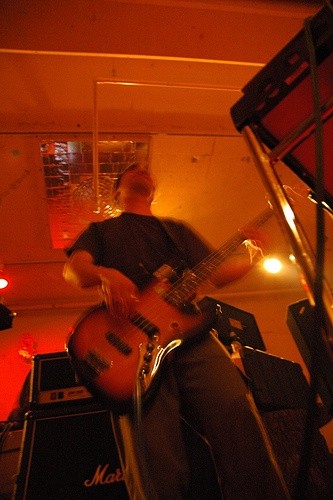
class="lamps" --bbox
[40,134,154,253]
[0,277,9,291]
[262,258,282,275]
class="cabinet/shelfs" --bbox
[196,296,268,350]
[187,344,332,500]
[10,351,133,500]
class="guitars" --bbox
[66,208,275,411]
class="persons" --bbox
[59,163,291,500]
